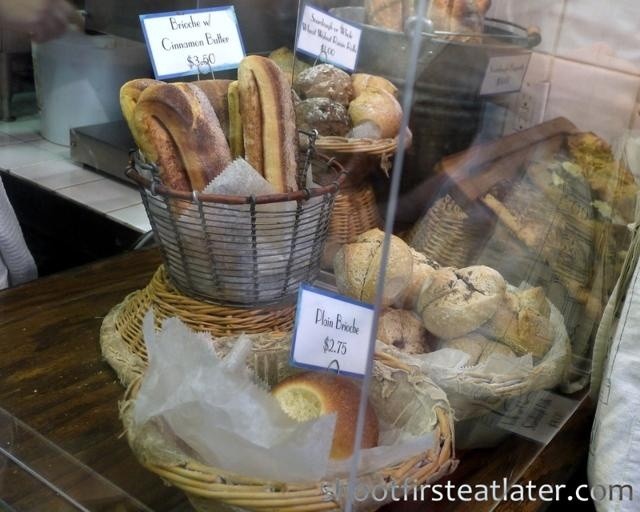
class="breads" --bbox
[119,46,640,367]
[267,372,379,459]
[364,0,492,42]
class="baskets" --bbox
[101,131,630,512]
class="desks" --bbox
[1,117,608,511]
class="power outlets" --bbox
[502,78,551,138]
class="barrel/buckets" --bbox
[328,6,543,201]
[30,32,157,147]
